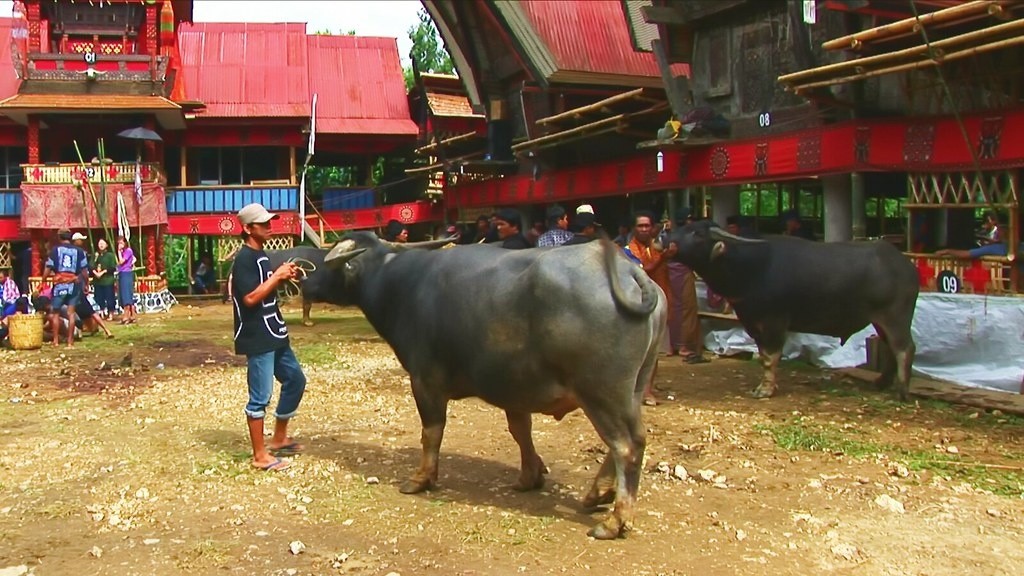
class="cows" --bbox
[301,229,671,543]
[660,217,922,406]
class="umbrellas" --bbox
[116,126,163,159]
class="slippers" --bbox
[682,353,711,364]
[273,438,304,451]
[252,458,291,471]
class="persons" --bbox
[231,202,307,471]
[936,210,1024,266]
[384,204,814,407]
[0,229,218,346]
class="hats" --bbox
[575,212,602,226]
[577,205,594,215]
[237,203,280,224]
[71,232,88,240]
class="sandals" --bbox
[116,319,137,325]
